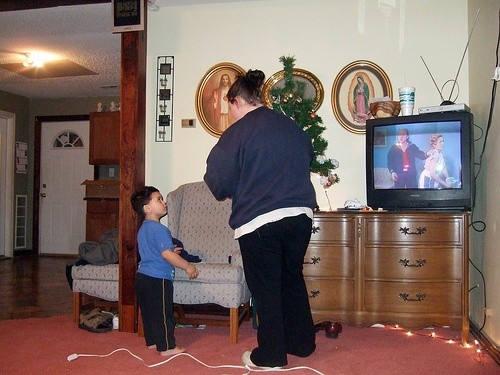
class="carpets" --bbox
[0,313,500,375]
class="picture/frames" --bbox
[331,60,393,134]
[195,62,247,138]
[262,69,324,113]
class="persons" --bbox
[131,186,199,356]
[387,128,452,189]
[203,69,320,369]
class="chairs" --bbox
[71,264,119,323]
[138,181,252,345]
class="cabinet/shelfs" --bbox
[86,201,120,244]
[16,196,27,250]
[254,211,471,346]
[88,112,120,164]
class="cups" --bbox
[398,86,416,116]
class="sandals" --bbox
[79,308,113,333]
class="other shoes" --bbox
[242,350,277,367]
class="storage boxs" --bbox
[80,180,120,198]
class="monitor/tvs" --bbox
[366,111,476,213]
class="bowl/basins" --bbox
[370,101,401,119]
